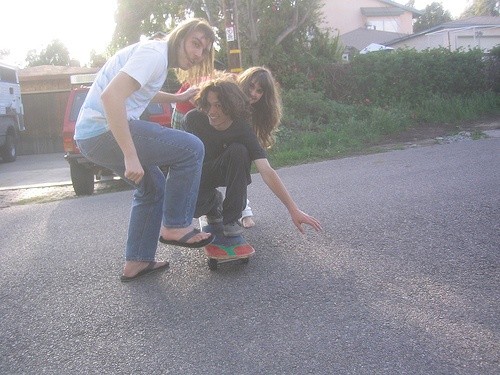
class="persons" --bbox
[73,19,217,282]
[169,67,322,237]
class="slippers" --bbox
[159,227,215,248]
[121,260,169,282]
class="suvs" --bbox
[0,64,28,162]
[60,72,175,195]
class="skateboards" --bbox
[199,211,256,271]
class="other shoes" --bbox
[237,199,255,228]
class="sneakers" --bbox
[207,192,224,224]
[223,222,244,237]
